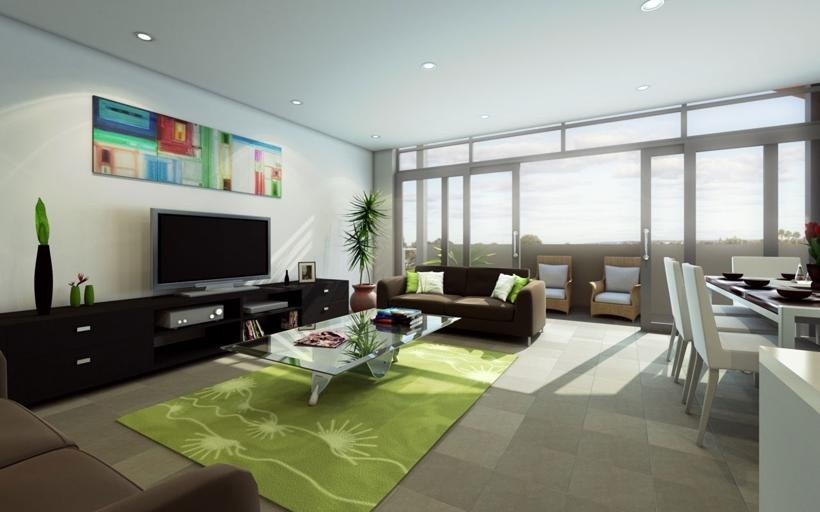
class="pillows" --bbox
[405,271,418,293]
[416,271,444,295]
[508,274,530,303]
[491,273,518,303]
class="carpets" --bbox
[115,340,518,512]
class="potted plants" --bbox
[337,188,392,312]
[68,273,88,305]
[33,199,52,311]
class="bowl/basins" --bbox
[722,273,812,299]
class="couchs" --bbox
[0,352,261,512]
[377,266,546,346]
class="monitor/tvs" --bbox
[149,206,273,299]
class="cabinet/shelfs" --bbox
[0,279,350,408]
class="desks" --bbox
[219,307,462,405]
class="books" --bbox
[243,319,265,340]
[376,307,423,328]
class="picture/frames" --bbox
[298,261,317,283]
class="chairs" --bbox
[586,256,642,322]
[663,256,819,450]
[534,255,573,316]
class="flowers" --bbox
[805,221,820,263]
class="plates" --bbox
[717,278,820,303]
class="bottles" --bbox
[284,270,290,285]
